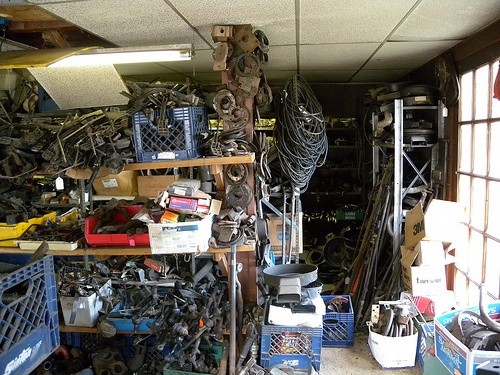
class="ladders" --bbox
[352,172,382,262]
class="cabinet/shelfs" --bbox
[0,152,255,335]
[208,123,366,248]
[371,97,446,299]
[186,167,300,274]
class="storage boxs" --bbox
[133,175,222,255]
[133,105,206,162]
[367,196,500,375]
[264,213,305,255]
[333,209,364,222]
[84,201,149,248]
[320,295,355,349]
[261,299,322,375]
[0,253,60,375]
[92,171,139,196]
[1,211,57,247]
[61,278,223,375]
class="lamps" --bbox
[48,43,197,64]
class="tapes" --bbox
[257,219,267,240]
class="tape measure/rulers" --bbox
[261,193,296,226]
